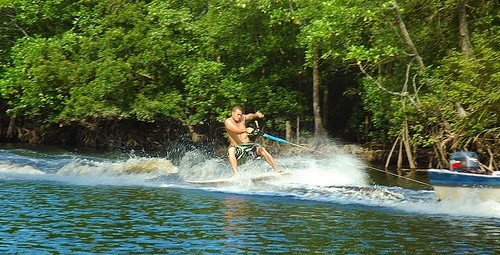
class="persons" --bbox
[224,106,283,178]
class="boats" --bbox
[426,151,500,212]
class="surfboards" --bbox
[184,171,291,183]
